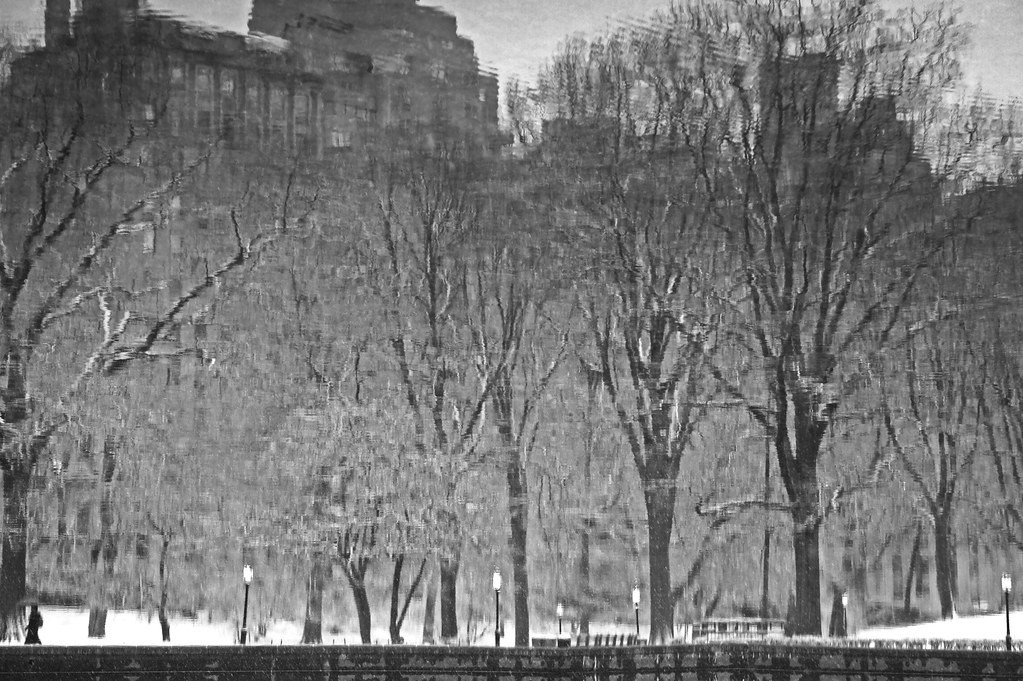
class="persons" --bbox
[24,606,43,644]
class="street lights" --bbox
[557,603,563,634]
[493,571,502,647]
[1001,571,1012,651]
[240,564,253,643]
[632,588,641,635]
[841,592,848,636]
